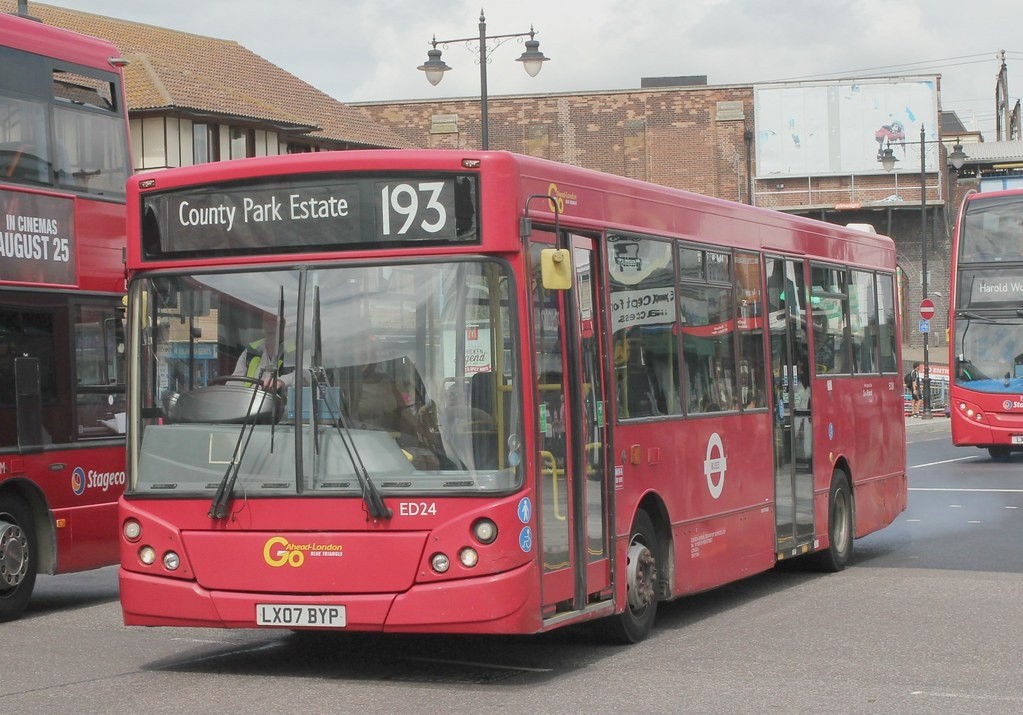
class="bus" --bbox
[1,17,160,625]
[946,186,1023,461]
[114,142,912,649]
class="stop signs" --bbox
[919,298,936,320]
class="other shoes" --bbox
[911,413,920,418]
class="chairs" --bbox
[469,371,509,444]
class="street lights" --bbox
[416,9,550,150]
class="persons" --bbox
[795,306,844,374]
[545,394,567,458]
[225,291,315,393]
[911,361,923,419]
[675,383,786,468]
[439,383,470,425]
[983,343,1010,367]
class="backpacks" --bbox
[904,370,916,389]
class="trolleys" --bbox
[927,376,949,415]
